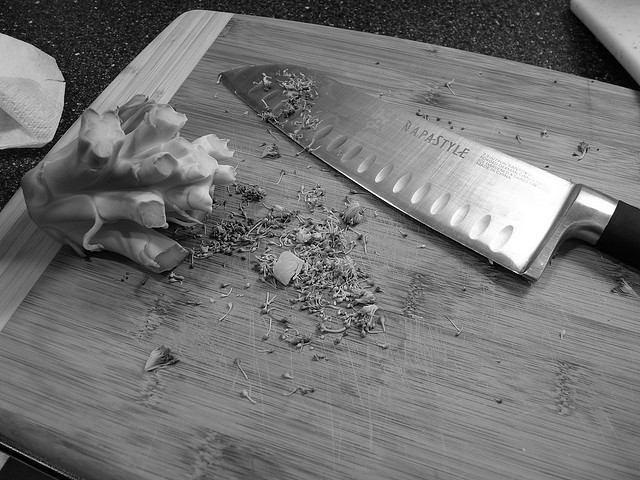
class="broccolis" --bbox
[20,94,238,274]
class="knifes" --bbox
[218,64,640,283]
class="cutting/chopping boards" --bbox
[0,7,639,476]
